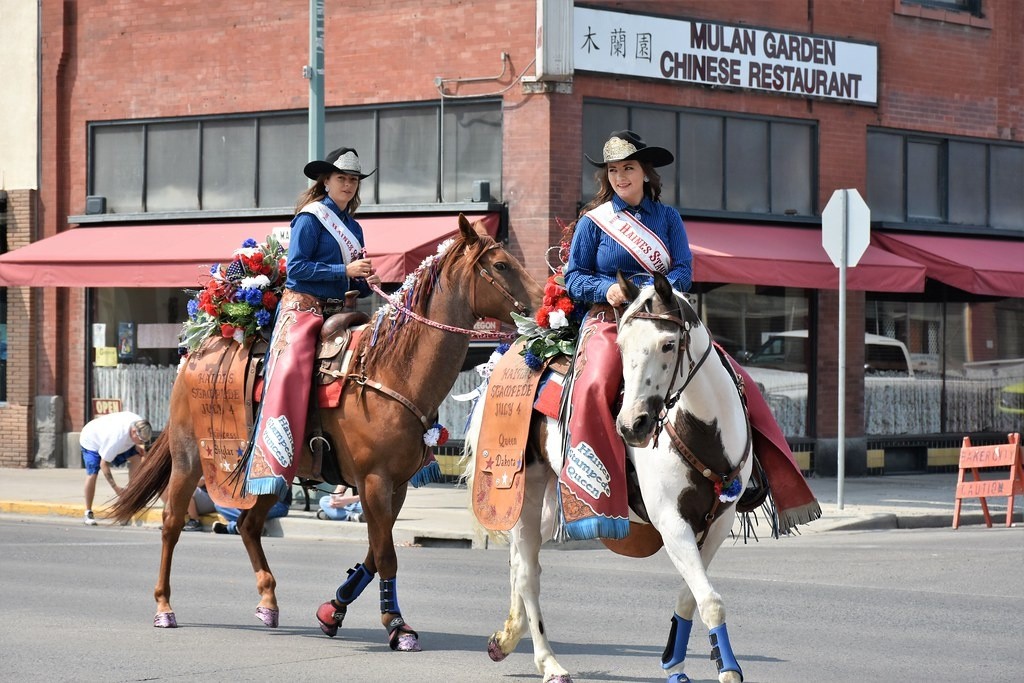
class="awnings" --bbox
[682,218,927,291]
[0,213,499,287]
[869,230,1024,298]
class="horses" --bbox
[458,270,753,683]
[94,213,545,652]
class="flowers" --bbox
[493,214,580,369]
[180,235,289,355]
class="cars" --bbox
[992,378,1024,428]
[733,328,917,411]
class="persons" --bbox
[247,146,382,503]
[80,411,152,524]
[557,130,822,541]
[182,475,216,531]
[316,485,367,522]
[212,486,293,534]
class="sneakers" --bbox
[235,525,241,535]
[158,525,164,530]
[181,519,202,531]
[211,521,228,534]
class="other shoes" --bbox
[84,510,97,525]
[316,509,330,521]
[352,513,367,523]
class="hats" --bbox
[587,129,674,168]
[302,147,378,181]
